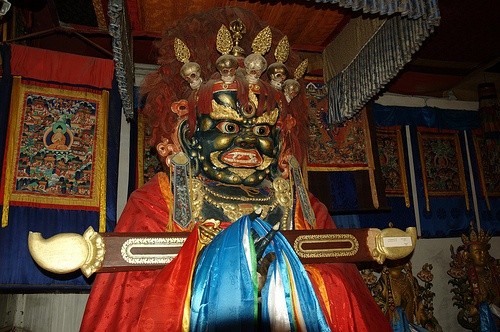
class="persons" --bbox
[361,227,500,332]
[79,7,391,332]
